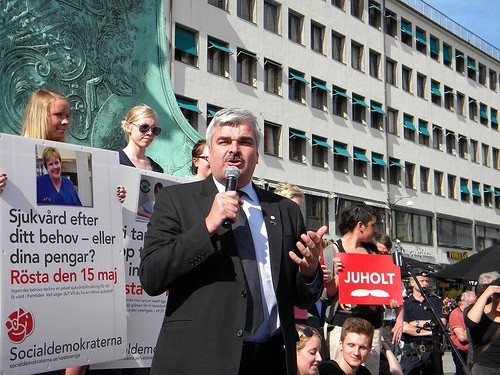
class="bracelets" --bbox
[416,320,419,327]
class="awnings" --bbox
[175,24,430,137]
[290,131,404,169]
[369,1,477,71]
[460,179,500,197]
[431,81,498,125]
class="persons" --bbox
[295,323,322,375]
[307,205,407,375]
[190,139,212,181]
[400,268,447,375]
[139,108,328,375]
[119,104,164,174]
[275,182,304,209]
[37,147,82,207]
[318,317,374,375]
[137,182,163,219]
[429,271,500,375]
[0,89,126,375]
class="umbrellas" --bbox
[431,238,500,286]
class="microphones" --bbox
[222,166,241,229]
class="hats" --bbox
[411,268,428,277]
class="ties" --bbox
[231,191,264,335]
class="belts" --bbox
[243,336,284,354]
[407,344,434,356]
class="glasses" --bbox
[131,122,162,136]
[378,250,391,255]
[299,326,318,338]
